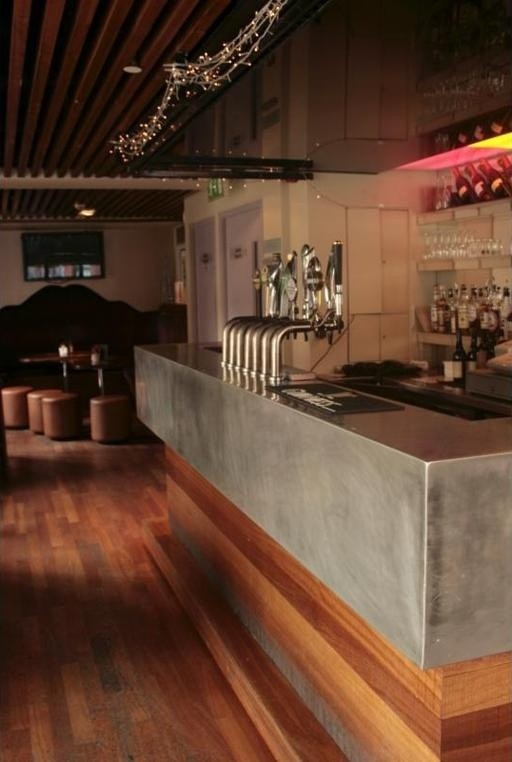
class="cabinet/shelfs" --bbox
[416,159,512,402]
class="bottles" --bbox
[455,104,512,148]
[431,280,510,332]
[451,331,480,384]
[452,155,511,203]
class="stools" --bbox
[41,394,81,440]
[90,395,130,443]
[1,386,33,429]
[26,389,61,433]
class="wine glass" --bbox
[433,175,452,211]
[422,229,477,258]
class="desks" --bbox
[60,356,123,395]
[19,351,90,393]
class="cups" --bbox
[441,360,455,383]
[433,132,453,155]
[468,236,503,256]
